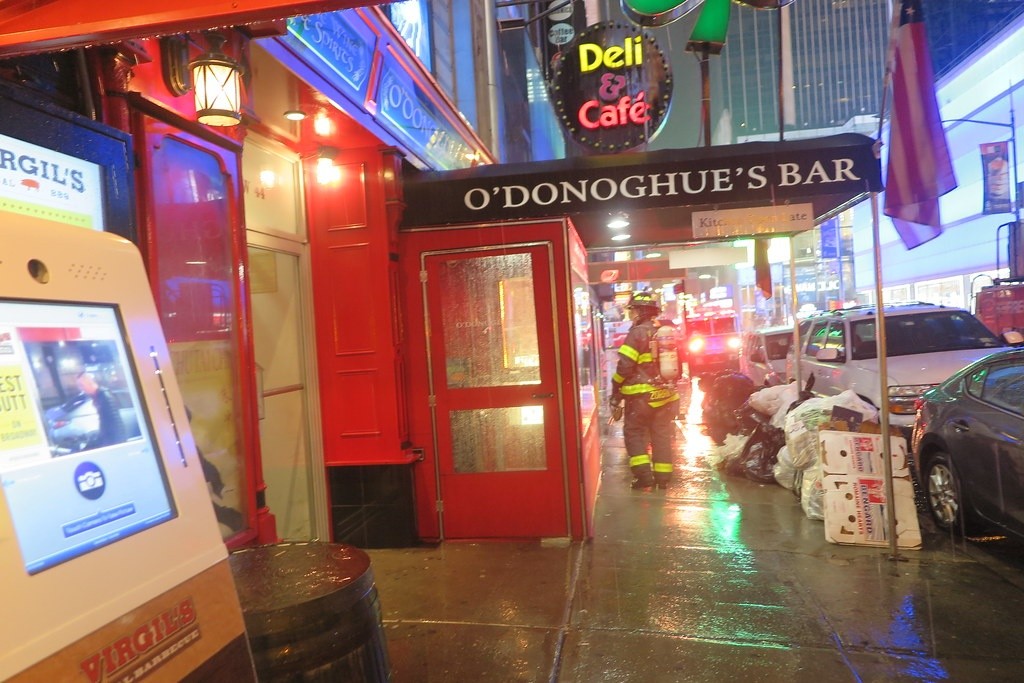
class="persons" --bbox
[609,293,680,489]
[74,371,127,451]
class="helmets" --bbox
[624,292,659,308]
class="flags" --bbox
[753,236,773,300]
[884,0,957,251]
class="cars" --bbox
[909,345,1024,538]
[739,326,795,387]
[786,301,1016,449]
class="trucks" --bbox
[684,312,742,375]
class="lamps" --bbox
[160,30,242,127]
[316,146,339,186]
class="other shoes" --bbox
[632,478,654,487]
[655,480,670,489]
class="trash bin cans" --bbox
[229,540,391,683]
